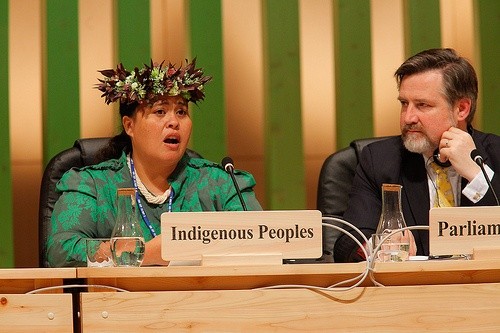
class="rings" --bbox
[446,140,448,147]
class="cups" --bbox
[86,238,111,267]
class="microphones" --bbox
[470,149,500,206]
[221,156,247,211]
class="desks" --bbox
[0,258,500,333]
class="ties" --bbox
[430,161,456,208]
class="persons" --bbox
[45,59,263,267]
[333,48,500,263]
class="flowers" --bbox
[95,57,214,106]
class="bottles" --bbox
[375,183,411,263]
[109,187,145,268]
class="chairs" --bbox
[317,136,394,261]
[38,137,204,268]
